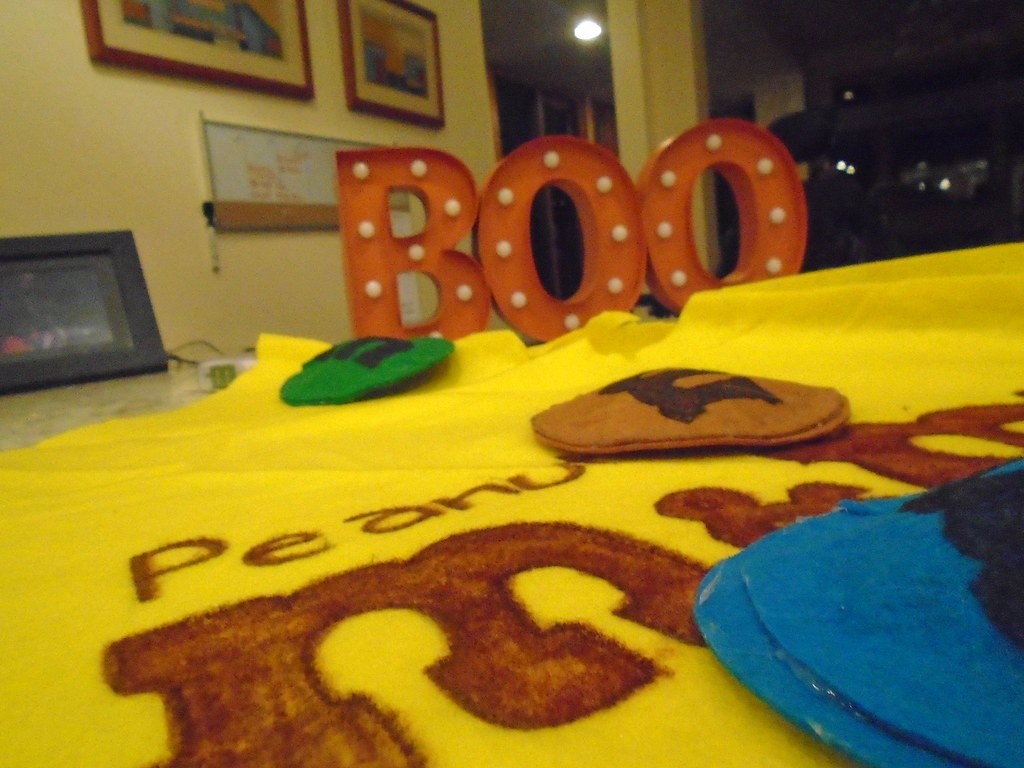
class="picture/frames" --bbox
[79,0,318,104]
[0,229,171,394]
[336,0,446,129]
